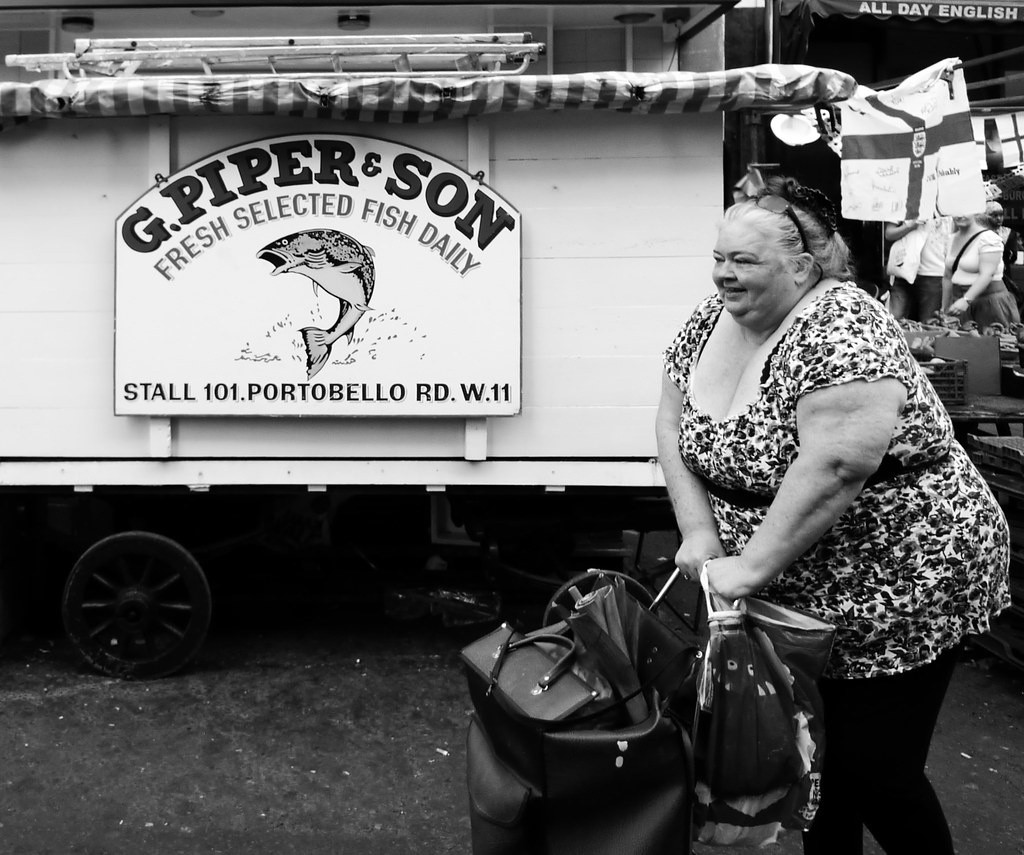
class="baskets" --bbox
[911,356,967,405]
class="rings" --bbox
[683,573,692,580]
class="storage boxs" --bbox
[911,352,969,403]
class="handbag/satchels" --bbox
[460,558,836,855]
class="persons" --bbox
[885,201,1024,330]
[654,174,1011,855]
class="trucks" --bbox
[0,33,856,679]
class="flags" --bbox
[838,57,987,220]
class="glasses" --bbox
[745,192,809,253]
